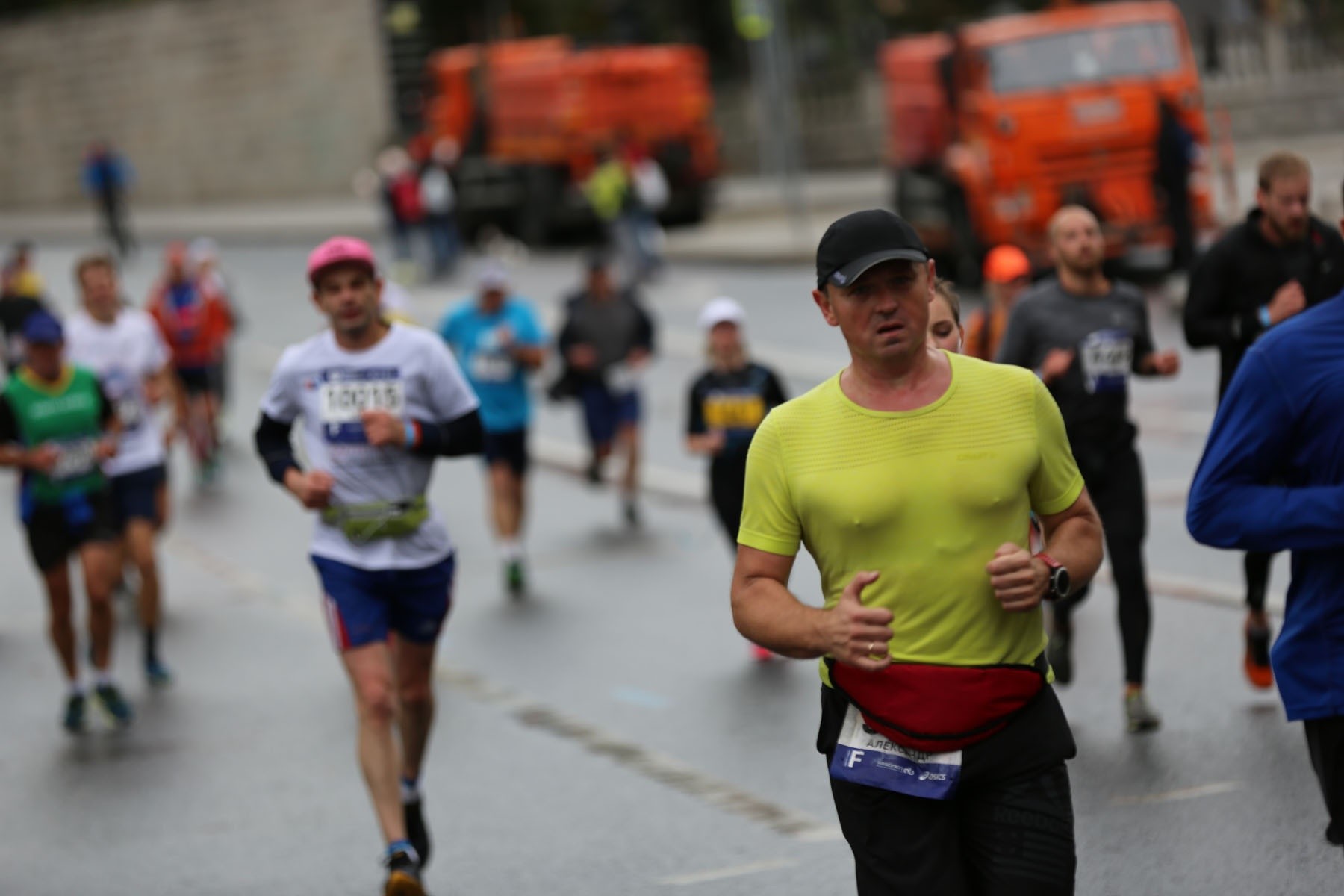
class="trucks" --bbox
[873,1,1222,292]
[411,33,720,251]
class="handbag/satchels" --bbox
[324,496,433,543]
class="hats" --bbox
[309,236,373,286]
[814,207,930,294]
[984,246,1030,285]
[701,298,744,333]
[24,314,62,345]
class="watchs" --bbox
[1036,551,1071,602]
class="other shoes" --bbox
[382,853,430,896]
[1244,619,1273,685]
[97,685,126,722]
[144,657,164,679]
[63,692,84,730]
[507,562,524,593]
[1047,628,1075,684]
[585,461,602,482]
[1123,690,1157,732]
[623,501,639,521]
[403,796,428,865]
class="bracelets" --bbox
[411,421,422,449]
[405,419,414,446]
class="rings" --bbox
[869,641,875,653]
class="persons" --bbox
[0,95,1343,896]
